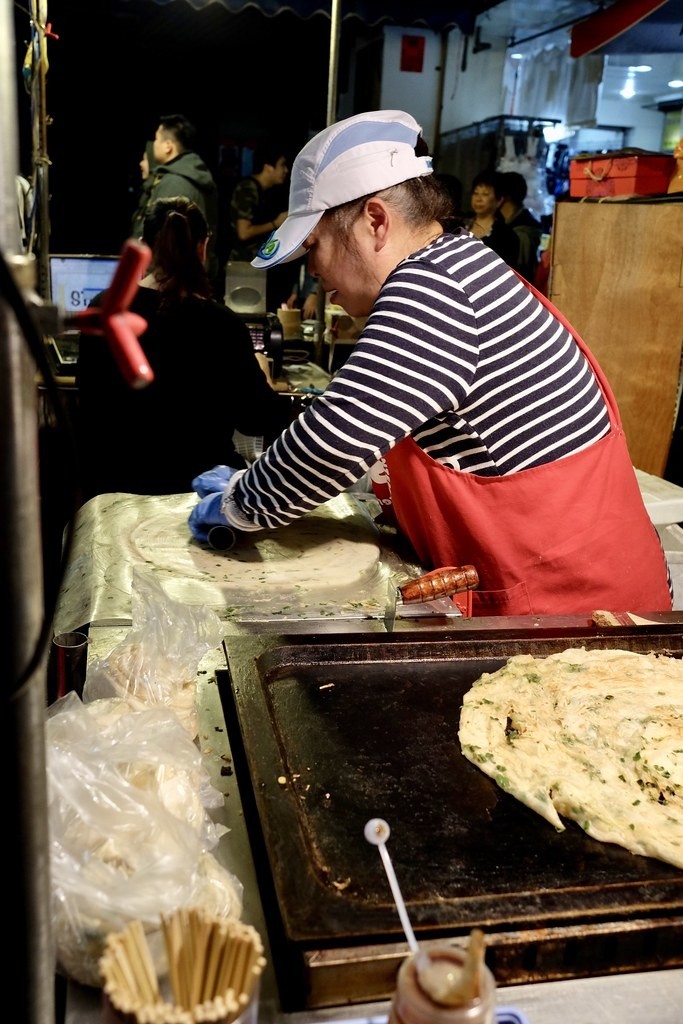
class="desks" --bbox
[33,338,333,452]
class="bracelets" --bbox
[271,221,277,229]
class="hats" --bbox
[250,109,433,271]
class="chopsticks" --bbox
[98,906,267,1024]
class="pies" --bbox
[458,648,683,870]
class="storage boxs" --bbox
[569,156,677,198]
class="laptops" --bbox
[46,254,124,367]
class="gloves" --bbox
[193,464,238,497]
[189,492,233,542]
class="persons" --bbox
[502,172,540,284]
[459,171,519,270]
[131,113,217,291]
[286,254,320,319]
[190,111,675,619]
[78,196,313,494]
[223,149,289,261]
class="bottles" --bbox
[388,945,496,1024]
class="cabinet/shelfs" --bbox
[546,194,683,478]
